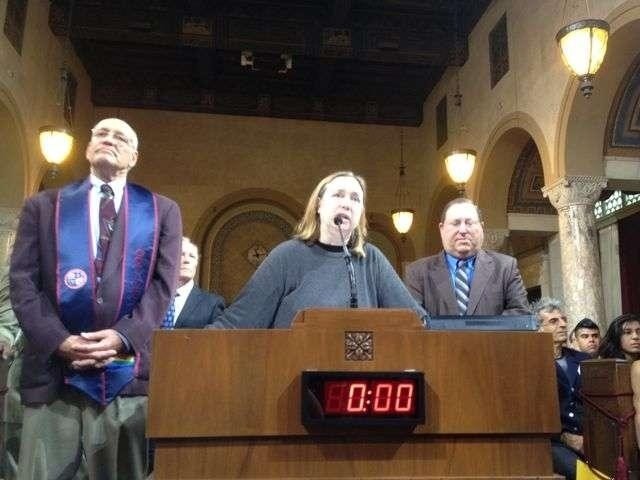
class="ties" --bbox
[93,185,117,290]
[454,258,470,318]
[162,292,178,329]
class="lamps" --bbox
[389,125,416,244]
[36,1,75,178]
[556,1,609,99]
[444,63,477,199]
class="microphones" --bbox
[334,215,358,308]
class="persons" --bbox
[7,116,184,479]
[203,171,428,330]
[403,197,533,316]
[158,236,225,329]
[574,317,601,357]
[529,295,591,480]
[568,330,581,352]
[598,312,640,361]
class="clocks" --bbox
[243,242,271,268]
